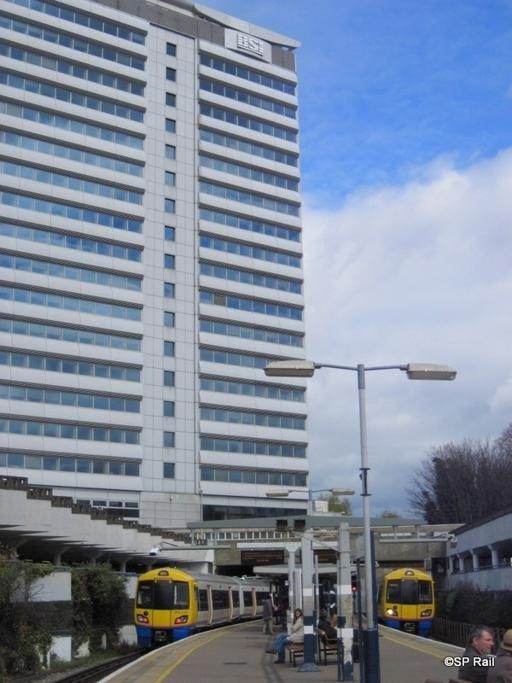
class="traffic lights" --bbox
[351,573,360,593]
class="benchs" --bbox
[449,678,472,683]
[316,628,337,665]
[287,645,304,666]
[425,679,445,683]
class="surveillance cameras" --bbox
[150,548,160,556]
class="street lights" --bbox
[261,356,460,682]
[264,484,356,672]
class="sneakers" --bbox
[265,649,276,654]
[274,659,285,664]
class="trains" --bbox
[132,565,280,653]
[377,565,439,640]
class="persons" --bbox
[318,611,338,646]
[263,594,278,635]
[458,625,494,683]
[486,629,512,683]
[265,608,304,663]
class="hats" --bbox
[499,628,512,651]
